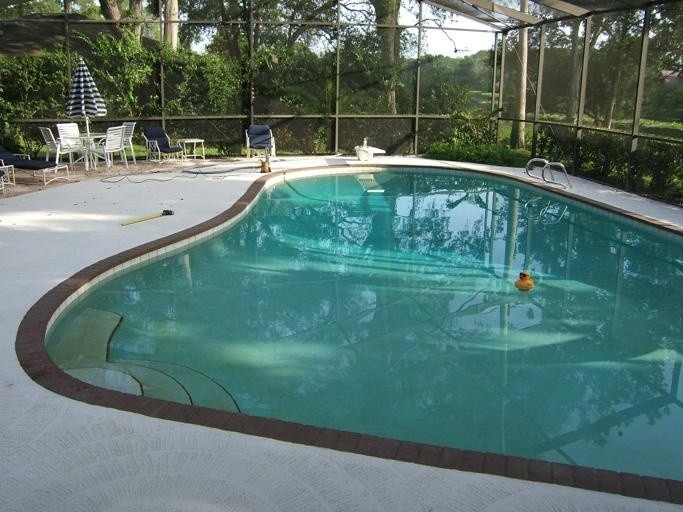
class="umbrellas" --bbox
[65,57,107,137]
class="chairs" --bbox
[245,124,276,160]
[37,118,183,168]
[1,146,69,195]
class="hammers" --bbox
[120,210,174,226]
